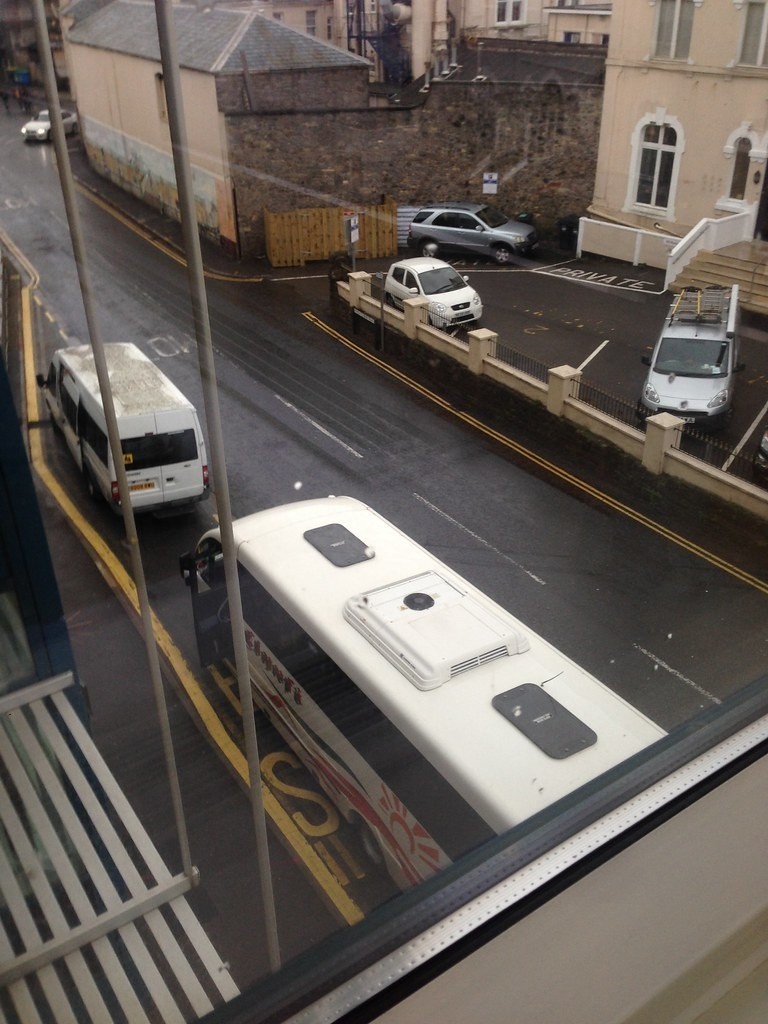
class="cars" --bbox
[383,257,483,328]
[21,109,79,143]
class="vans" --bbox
[36,340,211,516]
[637,284,745,433]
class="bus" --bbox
[178,495,668,895]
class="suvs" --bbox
[408,201,540,265]
